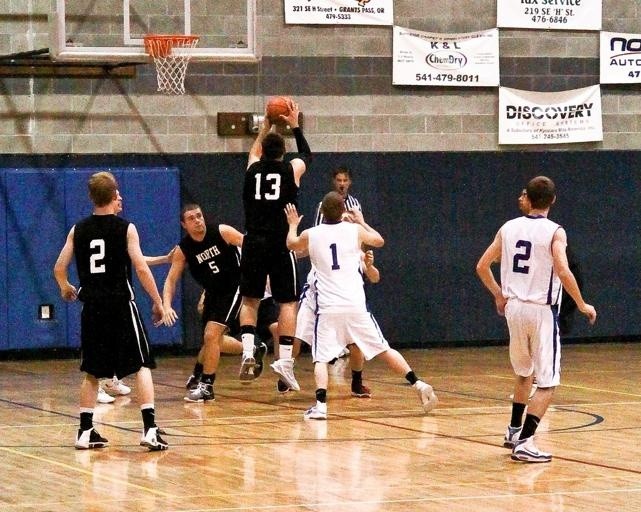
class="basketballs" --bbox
[266,97,292,125]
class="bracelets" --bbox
[492,288,503,297]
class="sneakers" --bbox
[504,424,552,463]
[411,380,438,412]
[351,386,372,398]
[140,424,169,450]
[239,342,301,392]
[96,376,131,403]
[304,407,327,420]
[184,375,215,403]
[75,426,110,449]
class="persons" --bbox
[284,191,440,420]
[93,190,177,402]
[237,100,313,390]
[509,187,586,400]
[476,175,598,463]
[54,172,168,452]
[315,168,363,365]
[163,203,379,402]
